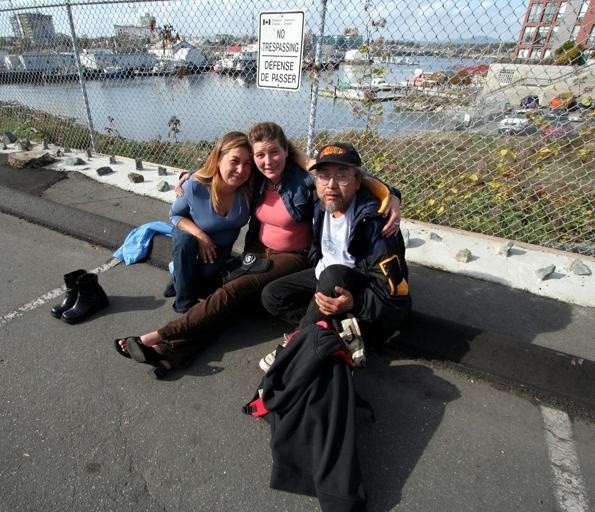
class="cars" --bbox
[495,91,594,136]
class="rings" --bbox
[394,222,400,229]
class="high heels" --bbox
[114,336,175,380]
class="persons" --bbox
[260,141,408,377]
[113,122,402,380]
[164,131,254,313]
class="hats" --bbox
[309,143,360,171]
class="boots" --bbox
[50,269,86,316]
[61,273,109,324]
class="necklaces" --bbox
[267,179,282,192]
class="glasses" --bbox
[315,173,353,186]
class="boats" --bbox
[1,35,486,98]
[316,47,409,103]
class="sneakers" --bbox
[259,343,286,373]
